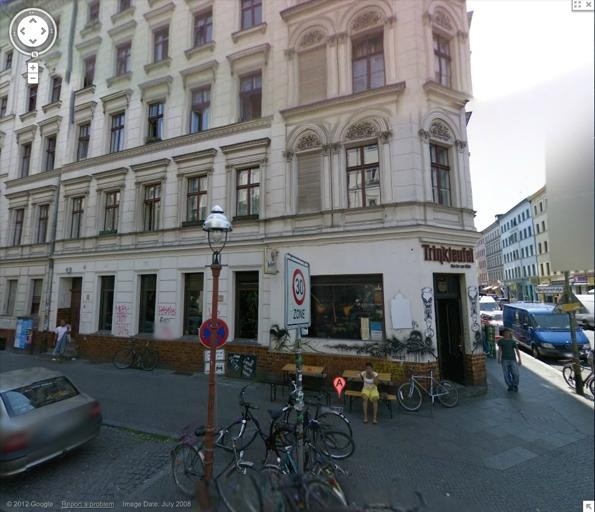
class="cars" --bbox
[0,366,103,479]
[571,308,595,329]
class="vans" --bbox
[478,295,505,333]
[503,301,591,362]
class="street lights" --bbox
[201,203,233,512]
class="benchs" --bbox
[343,389,397,410]
[262,379,317,402]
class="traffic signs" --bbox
[283,252,312,329]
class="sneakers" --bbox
[514,385,518,392]
[508,386,513,391]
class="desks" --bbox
[341,369,393,410]
[280,363,325,403]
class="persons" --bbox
[346,361,383,425]
[496,329,522,393]
[49,318,77,363]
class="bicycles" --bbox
[170,381,356,512]
[396,367,458,412]
[111,340,159,371]
[563,355,595,396]
